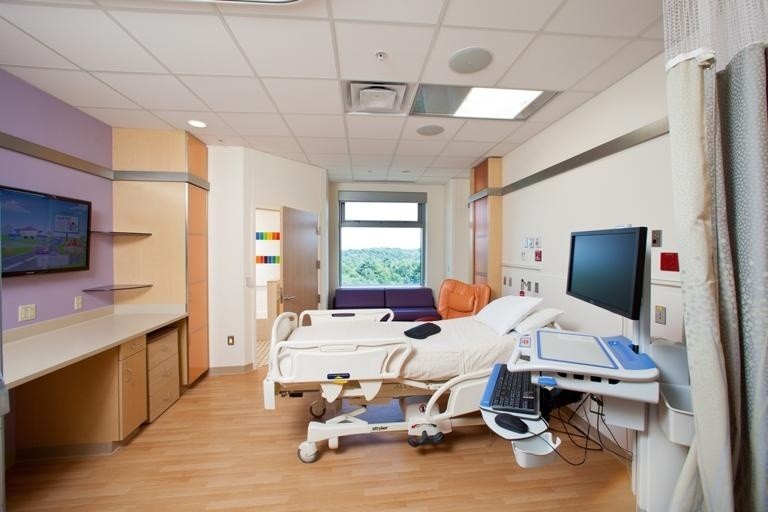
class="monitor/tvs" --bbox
[564,226,647,320]
[0,183,92,277]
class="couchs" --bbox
[333,287,437,320]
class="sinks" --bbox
[658,383,696,448]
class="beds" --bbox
[264,320,561,465]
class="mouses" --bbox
[494,413,528,434]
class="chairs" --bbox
[415,279,491,322]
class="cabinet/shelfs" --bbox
[113,128,210,442]
[147,327,181,424]
[9,332,146,454]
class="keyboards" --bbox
[491,364,537,414]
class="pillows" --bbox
[470,295,563,336]
